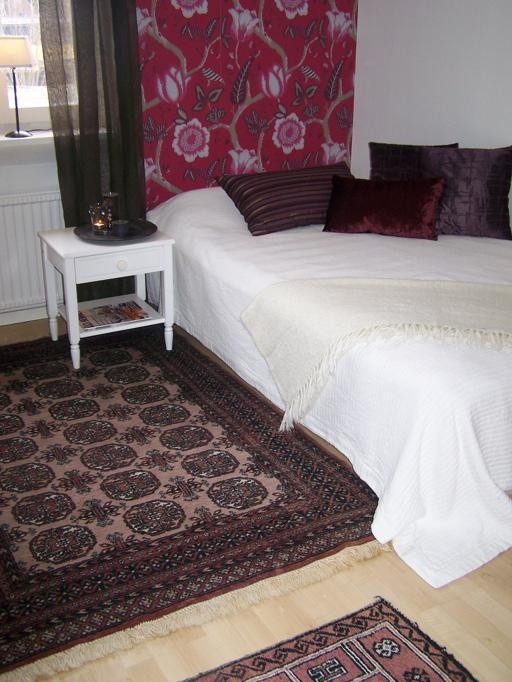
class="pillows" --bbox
[215,142,511,241]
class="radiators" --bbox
[1,191,66,312]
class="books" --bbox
[78,300,152,331]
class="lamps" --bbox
[1,35,36,138]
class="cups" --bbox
[88,202,114,237]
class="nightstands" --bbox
[37,227,175,370]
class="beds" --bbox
[144,175,511,508]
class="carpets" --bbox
[178,596,480,682]
[0,321,377,675]
